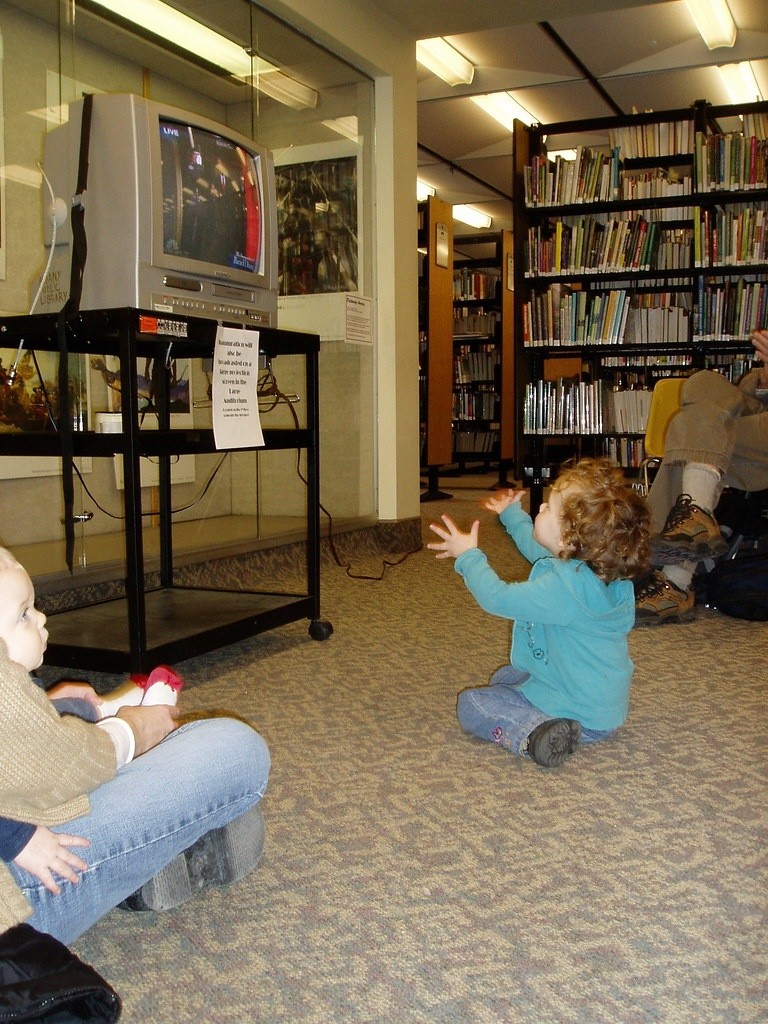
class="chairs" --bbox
[635,459,768,608]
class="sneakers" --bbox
[636,494,730,568]
[631,578,696,628]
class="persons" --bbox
[426,457,651,768]
[0,545,273,949]
[632,330,768,629]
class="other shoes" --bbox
[117,801,265,911]
[528,717,581,767]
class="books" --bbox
[522,131,768,466]
[419,266,500,454]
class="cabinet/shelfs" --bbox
[418,101,768,527]
[1,306,334,679]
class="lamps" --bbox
[684,0,737,52]
[416,37,475,88]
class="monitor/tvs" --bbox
[40,91,280,328]
